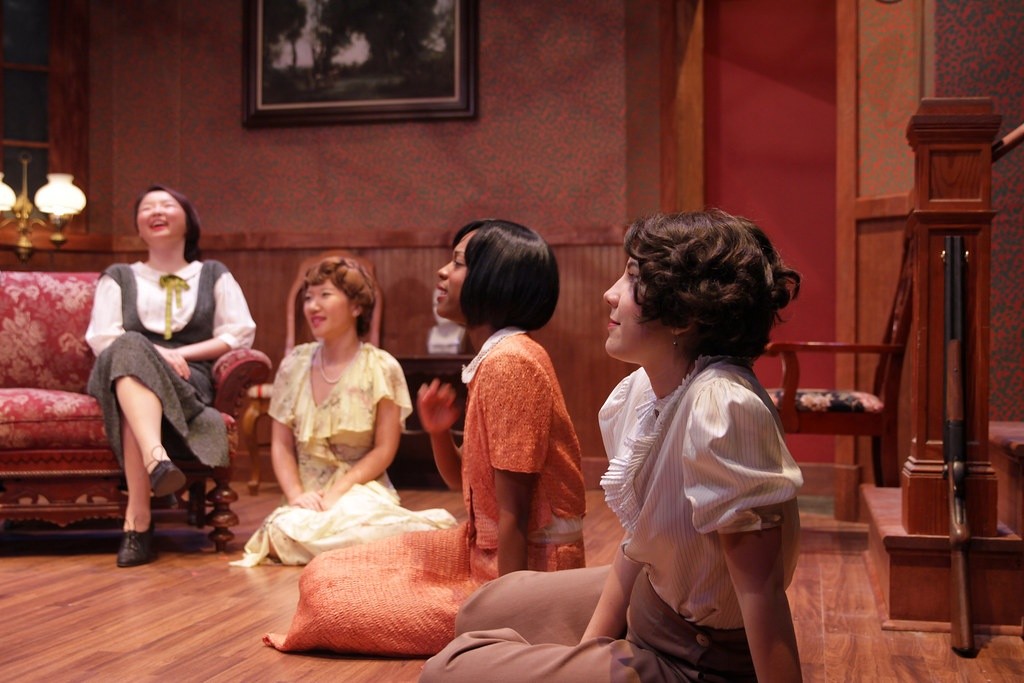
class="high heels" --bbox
[144,445,187,497]
[116,513,156,567]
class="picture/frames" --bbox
[240,0,480,130]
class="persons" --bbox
[260,216,588,656]
[226,255,460,570]
[419,206,806,683]
[84,184,257,569]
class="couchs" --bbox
[0,271,274,552]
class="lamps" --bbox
[0,150,88,270]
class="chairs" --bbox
[245,249,384,496]
[765,207,916,488]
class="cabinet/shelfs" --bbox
[390,353,475,493]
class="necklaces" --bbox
[319,340,364,384]
